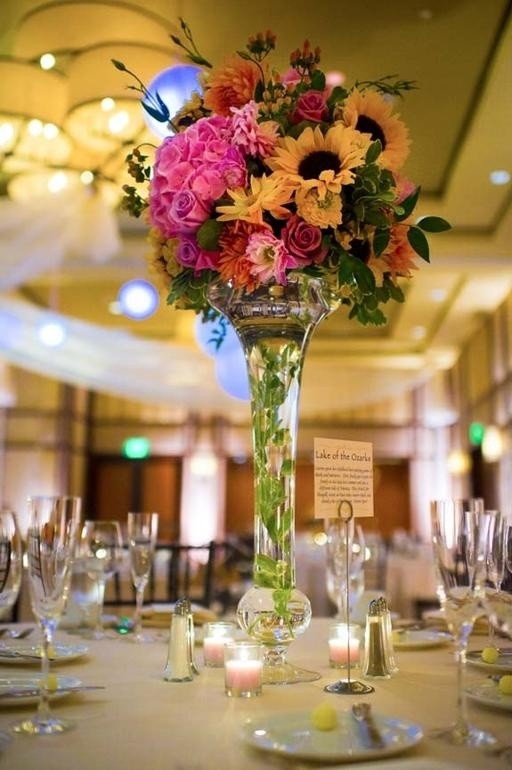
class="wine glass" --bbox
[122,511,159,645]
[322,513,365,632]
[426,495,512,749]
[0,495,123,738]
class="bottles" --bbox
[362,594,401,682]
[161,595,200,684]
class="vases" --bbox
[202,275,341,687]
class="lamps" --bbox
[1,0,189,216]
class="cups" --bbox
[201,620,239,670]
[326,623,363,670]
[220,641,262,699]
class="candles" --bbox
[200,621,362,697]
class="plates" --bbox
[390,626,456,649]
[458,646,512,672]
[463,684,512,712]
[235,708,423,763]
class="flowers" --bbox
[107,14,452,645]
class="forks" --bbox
[351,703,372,748]
